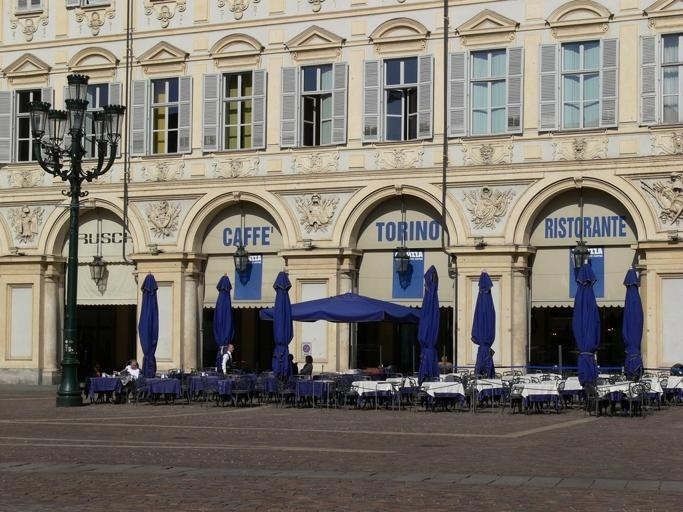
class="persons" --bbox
[85,364,111,403]
[299,355,313,379]
[108,360,140,404]
[220,343,235,379]
[287,354,296,368]
[295,192,340,232]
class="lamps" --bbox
[232,244,248,278]
[571,240,590,271]
[89,255,105,286]
[392,246,409,279]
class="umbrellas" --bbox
[571,259,602,387]
[418,264,440,384]
[271,268,293,377]
[258,290,420,370]
[470,269,495,379]
[622,264,643,380]
[212,271,234,372]
[138,271,158,378]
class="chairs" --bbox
[85,363,683,419]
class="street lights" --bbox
[28,74,127,408]
